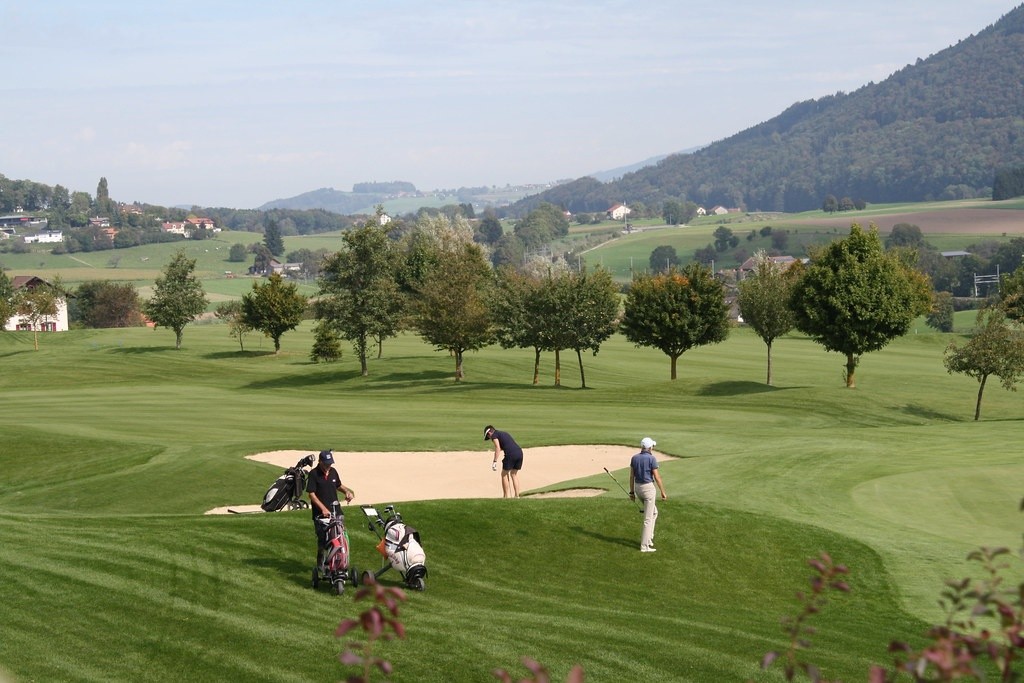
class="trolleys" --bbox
[358,503,429,592]
[272,455,315,512]
[313,511,360,596]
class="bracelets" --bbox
[629,492,634,494]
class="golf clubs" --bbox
[330,500,344,519]
[376,505,400,529]
[294,454,315,469]
[507,471,512,497]
[604,467,644,513]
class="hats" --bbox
[320,449,334,464]
[483,428,490,440]
[641,437,656,448]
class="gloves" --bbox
[492,462,497,471]
[629,492,636,501]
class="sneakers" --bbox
[641,548,657,552]
[649,541,654,546]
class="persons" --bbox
[305,451,353,575]
[484,425,523,498]
[629,437,666,552]
